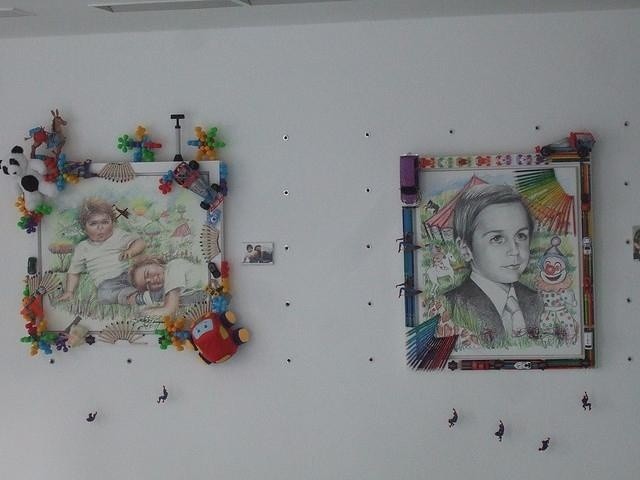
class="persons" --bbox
[55,200,144,305]
[441,184,540,344]
[127,258,208,316]
[244,245,273,263]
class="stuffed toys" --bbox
[1,146,59,213]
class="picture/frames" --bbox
[399,146,597,370]
[31,159,225,348]
